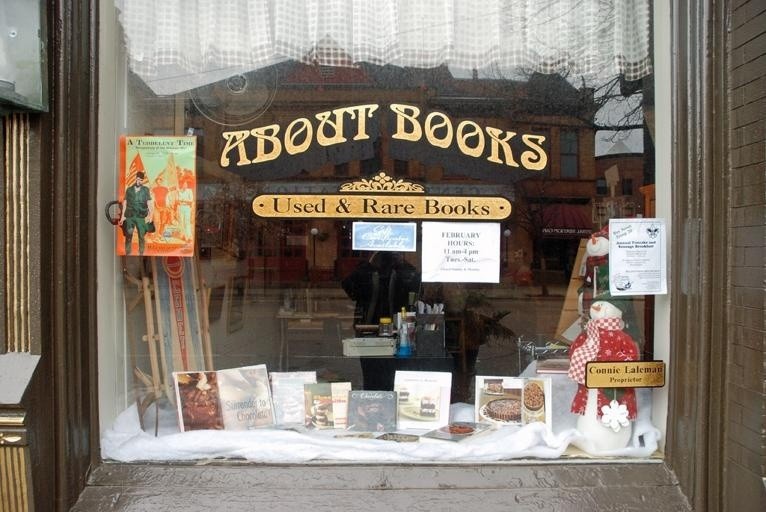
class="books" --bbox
[473,375,554,431]
[173,362,453,435]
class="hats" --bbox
[136,172,144,180]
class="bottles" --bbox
[396,306,413,357]
[378,317,394,337]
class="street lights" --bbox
[501,228,514,270]
[310,226,319,268]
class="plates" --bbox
[522,381,545,418]
[400,404,439,422]
[479,402,522,425]
[438,424,477,436]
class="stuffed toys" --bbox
[577,224,642,352]
[568,292,643,420]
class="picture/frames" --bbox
[205,273,250,336]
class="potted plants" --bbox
[449,290,518,376]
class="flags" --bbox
[125,153,149,189]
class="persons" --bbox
[119,172,154,255]
[341,236,421,390]
[176,181,192,242]
[151,178,170,240]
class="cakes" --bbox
[486,399,522,420]
[182,372,218,427]
[524,383,544,410]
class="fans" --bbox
[188,64,280,130]
